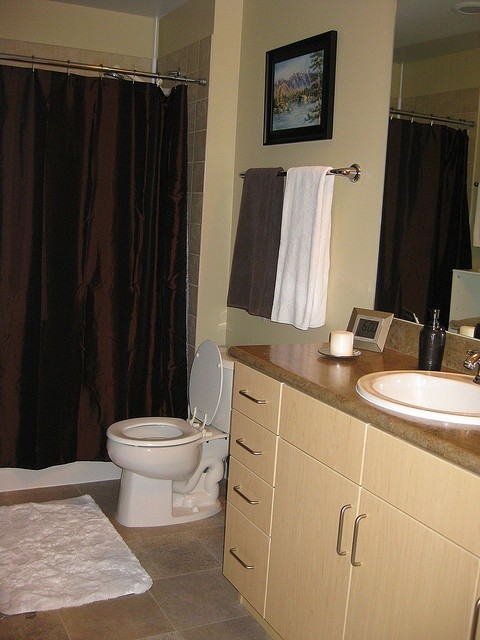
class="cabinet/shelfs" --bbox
[267,386,480,639]
[218,360,283,619]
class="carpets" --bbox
[0,494,153,615]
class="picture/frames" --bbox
[262,29,337,148]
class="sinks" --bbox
[355,369,480,425]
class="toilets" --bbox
[107,340,228,527]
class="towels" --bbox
[225,166,286,320]
[268,165,334,332]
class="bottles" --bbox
[419,308,446,371]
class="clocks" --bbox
[346,309,395,352]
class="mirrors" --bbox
[372,1,479,341]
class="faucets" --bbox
[461,348,480,385]
